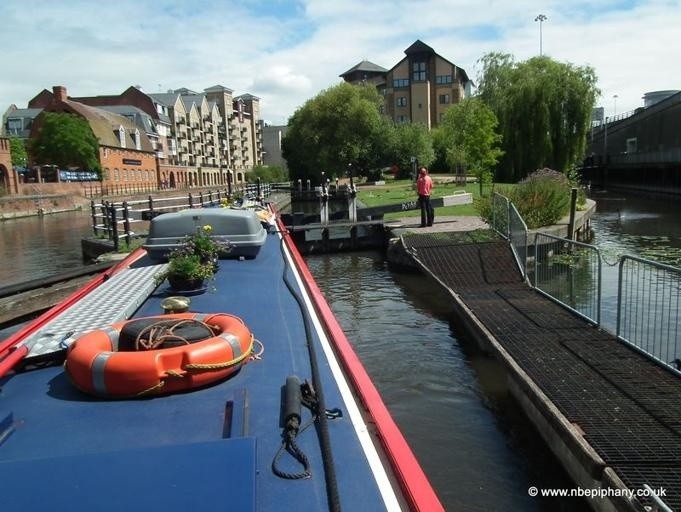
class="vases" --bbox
[164,279,209,297]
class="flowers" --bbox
[152,224,237,293]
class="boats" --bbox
[0,191,451,511]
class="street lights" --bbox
[613,94,619,114]
[535,13,548,57]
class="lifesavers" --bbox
[392,165,398,173]
[67,313,254,399]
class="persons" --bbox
[416,167,433,227]
